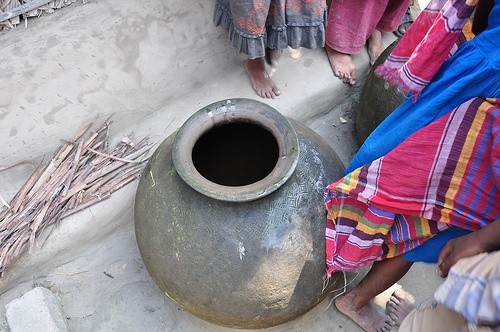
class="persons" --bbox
[211,0,330,100]
[333,0,500,332]
[325,0,412,85]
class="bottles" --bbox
[134,98,350,329]
